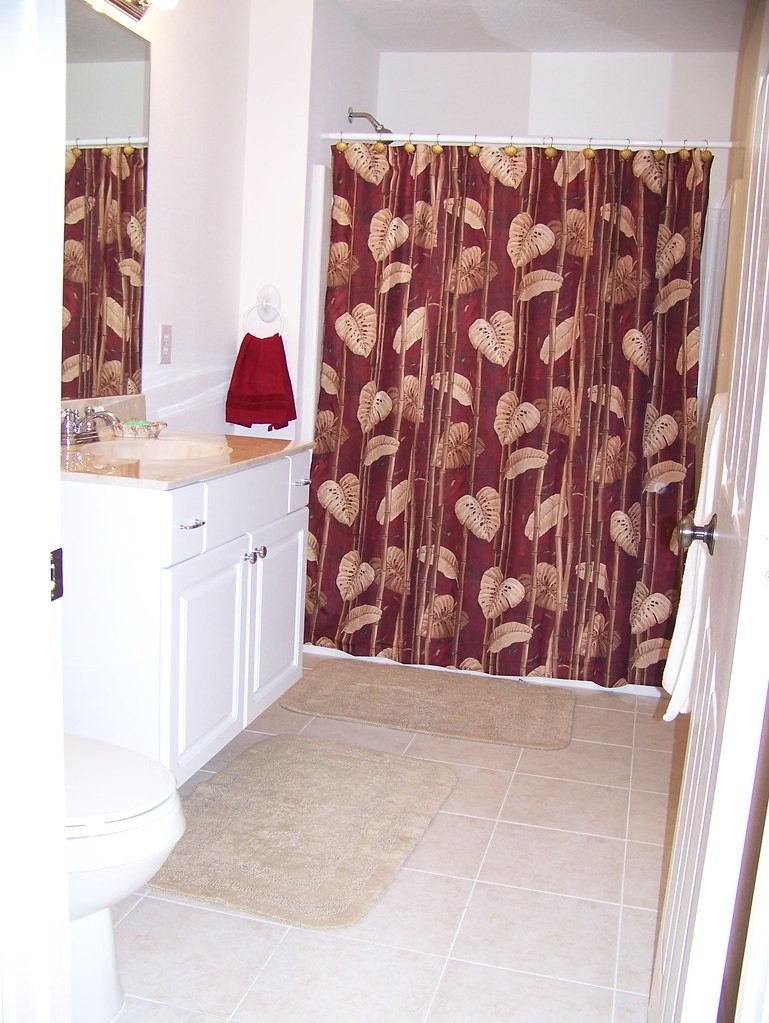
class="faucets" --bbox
[73,411,122,442]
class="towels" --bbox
[224,332,299,431]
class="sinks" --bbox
[82,438,234,460]
